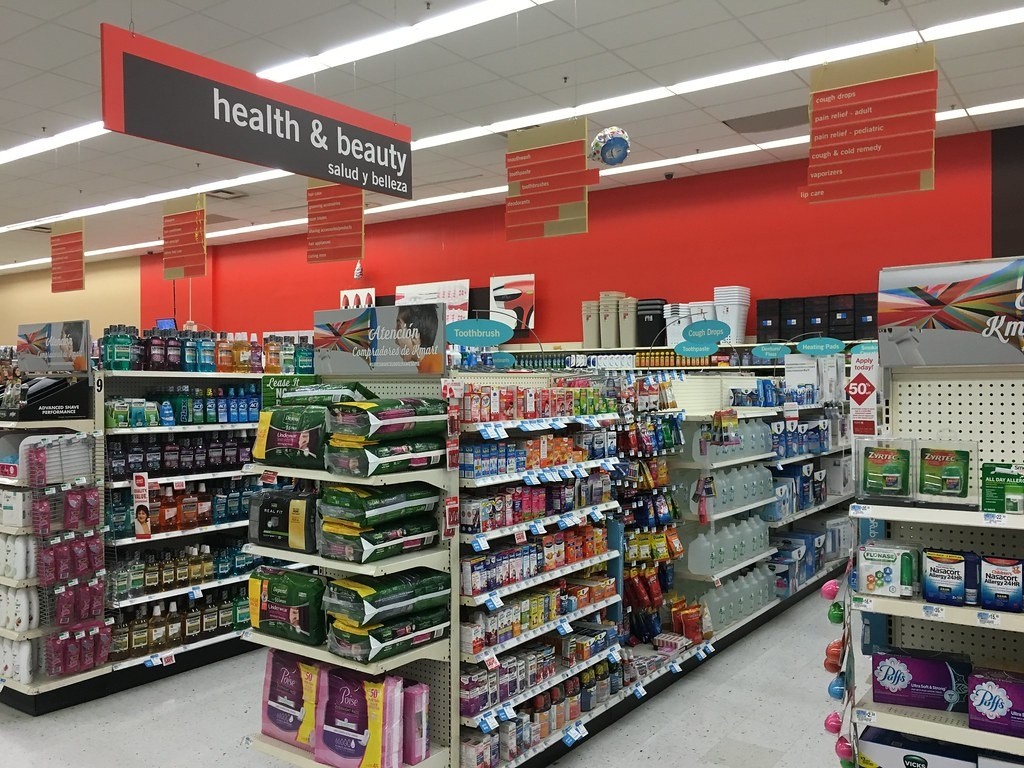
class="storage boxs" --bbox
[461,378,1024,768]
[0,378,89,422]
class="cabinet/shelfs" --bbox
[668,406,782,652]
[104,369,284,692]
[0,370,114,718]
[729,406,858,612]
[240,370,461,768]
[847,255,1024,758]
[461,412,628,768]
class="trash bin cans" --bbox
[582,285,878,349]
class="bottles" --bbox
[515,646,637,740]
[558,578,569,615]
[92,324,317,661]
[678,418,777,632]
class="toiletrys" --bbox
[102,323,314,664]
[0,486,47,684]
